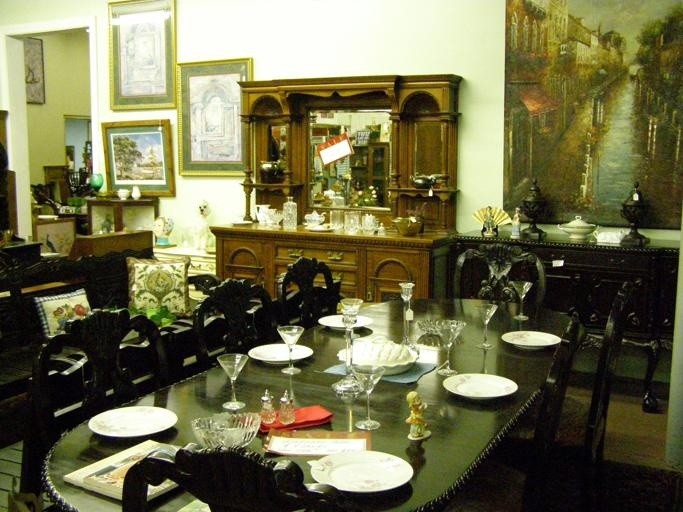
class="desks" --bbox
[87,196,160,236]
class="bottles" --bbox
[260,162,279,183]
[117,189,129,200]
[131,186,139,199]
[259,388,276,425]
[282,196,297,231]
[280,390,294,425]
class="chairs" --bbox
[501,279,636,512]
[433,308,583,512]
[30,309,176,478]
[192,277,279,372]
[276,255,342,333]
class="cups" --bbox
[303,209,385,233]
[264,209,282,228]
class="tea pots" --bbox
[254,204,270,225]
[408,174,436,189]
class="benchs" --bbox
[1,246,226,448]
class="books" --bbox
[61,438,187,502]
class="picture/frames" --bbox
[100,120,177,198]
[106,1,178,110]
[24,36,47,104]
[174,58,255,178]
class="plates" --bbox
[416,318,436,335]
[87,407,177,439]
[501,329,559,353]
[248,344,313,366]
[309,449,415,493]
[317,313,371,330]
[442,372,518,401]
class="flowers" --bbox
[391,201,430,228]
[257,159,288,177]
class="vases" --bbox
[260,174,285,184]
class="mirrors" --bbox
[262,122,287,163]
[302,105,393,211]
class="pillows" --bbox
[32,289,93,339]
[125,254,193,317]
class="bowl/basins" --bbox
[192,413,260,452]
[337,341,420,376]
[557,216,597,240]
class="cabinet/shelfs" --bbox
[452,227,680,415]
[347,138,388,210]
[210,227,451,307]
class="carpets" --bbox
[434,437,683,510]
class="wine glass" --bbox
[331,297,365,397]
[90,174,103,198]
[512,281,534,321]
[352,364,383,431]
[475,304,499,350]
[399,282,414,312]
[432,321,467,376]
[216,354,248,411]
[276,325,304,375]
